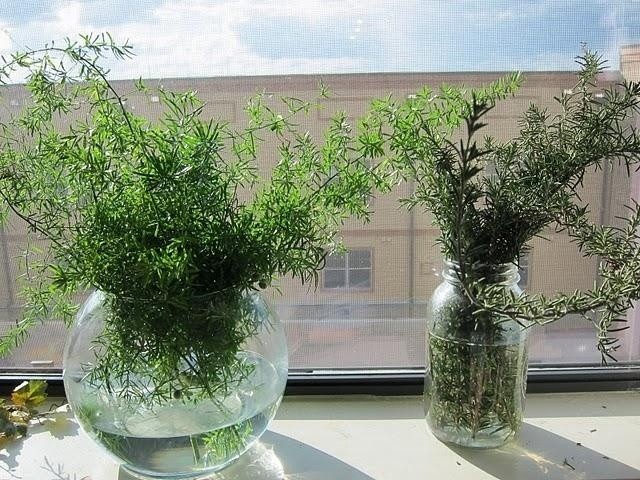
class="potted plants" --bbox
[0,33,523,480]
[376,40,640,450]
